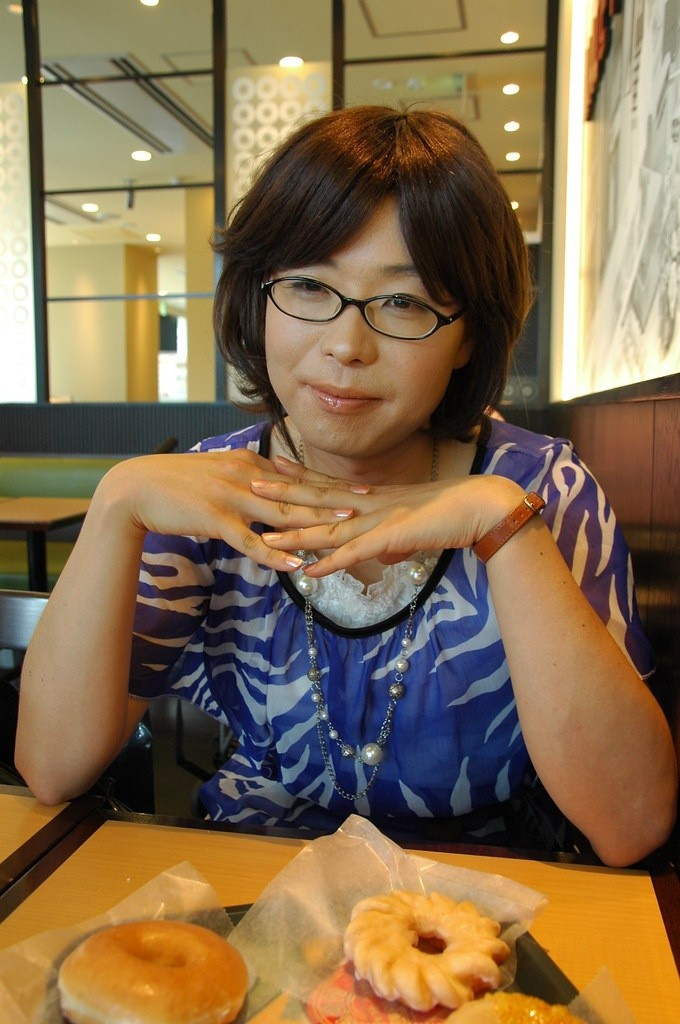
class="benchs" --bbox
[0,452,160,592]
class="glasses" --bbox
[260,277,465,341]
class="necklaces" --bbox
[299,438,439,800]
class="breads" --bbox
[58,920,248,1024]
[443,991,589,1024]
[343,889,510,1011]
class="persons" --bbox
[14,107,679,868]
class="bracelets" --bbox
[472,491,546,564]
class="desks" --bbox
[0,495,95,592]
[0,784,680,1023]
